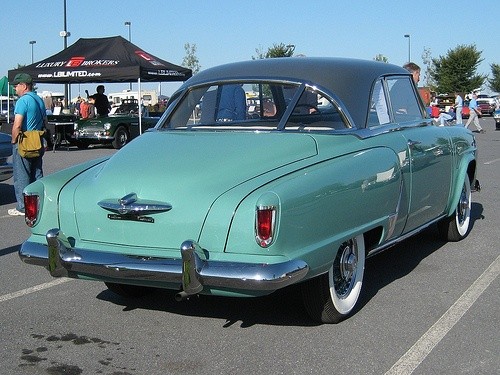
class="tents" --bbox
[0,36,199,135]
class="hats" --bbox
[10,73,32,85]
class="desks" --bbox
[49,121,73,153]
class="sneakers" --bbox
[8,207,25,216]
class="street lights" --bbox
[404,35,411,64]
[124,21,132,91]
[29,40,36,65]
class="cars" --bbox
[461,93,500,127]
[72,103,164,150]
[19,56,482,325]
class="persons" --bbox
[50,85,168,119]
[8,73,48,216]
[216,81,246,122]
[387,62,487,134]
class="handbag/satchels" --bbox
[17,130,45,158]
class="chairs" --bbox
[444,115,455,127]
[201,87,246,123]
